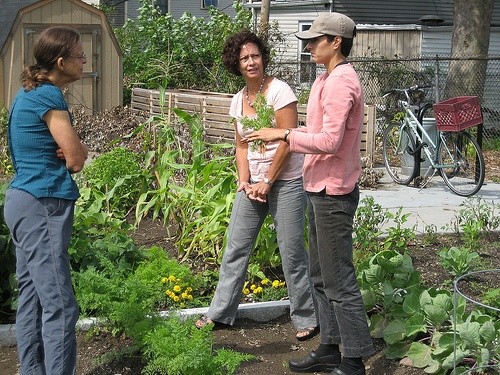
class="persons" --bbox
[194,31,320,342]
[239,13,375,375]
[3,27,89,375]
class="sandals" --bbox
[296,325,320,340]
[195,314,215,330]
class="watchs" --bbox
[283,129,292,141]
[263,178,272,186]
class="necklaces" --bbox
[246,76,265,107]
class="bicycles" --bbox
[380,83,485,197]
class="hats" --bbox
[295,12,357,40]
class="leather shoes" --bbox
[289,349,341,372]
[327,364,366,375]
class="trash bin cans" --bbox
[401,118,442,177]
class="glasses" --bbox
[68,52,86,59]
